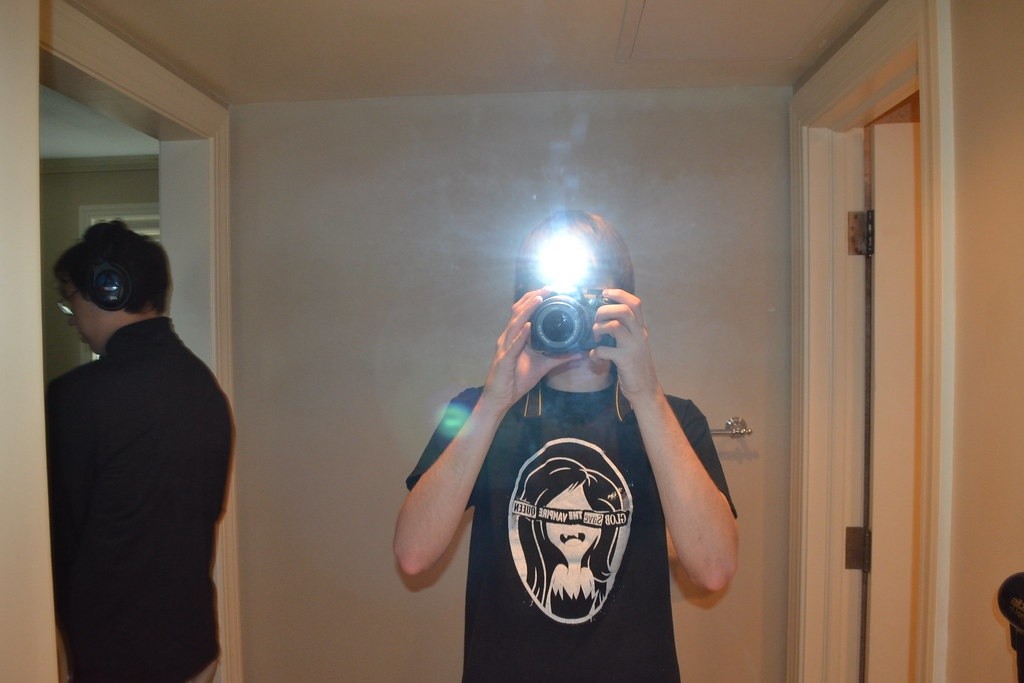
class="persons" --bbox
[44,220,233,682]
[394,209,738,683]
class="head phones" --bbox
[89,219,135,313]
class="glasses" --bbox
[56,289,78,316]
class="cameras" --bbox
[531,288,615,353]
